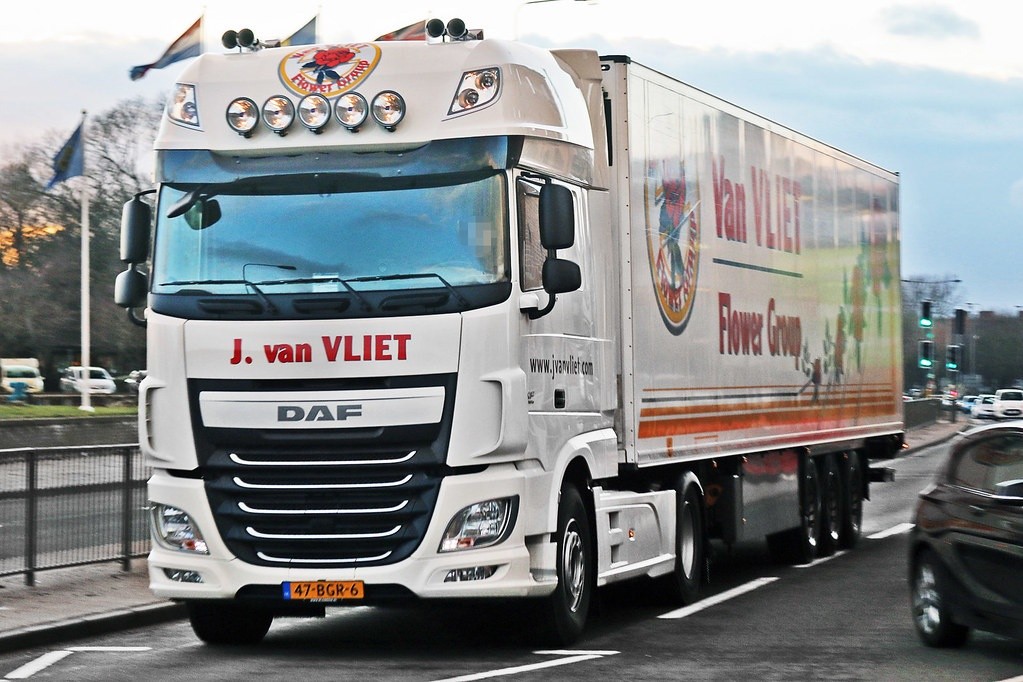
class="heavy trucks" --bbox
[114,18,906,644]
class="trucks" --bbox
[0,358,46,395]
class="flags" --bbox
[280,16,316,46]
[128,13,204,81]
[48,122,82,187]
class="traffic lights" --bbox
[955,309,966,334]
[918,340,932,368]
[947,346,959,372]
[919,302,932,328]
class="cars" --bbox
[970,394,995,419]
[990,389,1023,421]
[962,396,978,413]
[59,366,117,394]
[908,421,1023,650]
